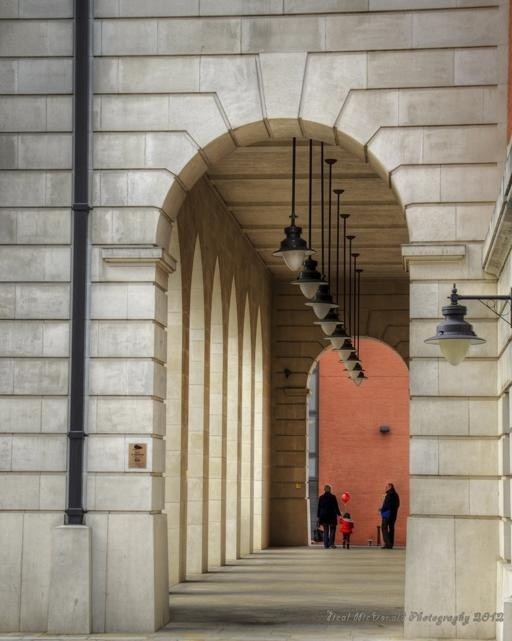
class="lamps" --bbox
[425,283,509,366]
[275,134,368,389]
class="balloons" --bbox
[340,492,349,507]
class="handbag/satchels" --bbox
[314,528,323,542]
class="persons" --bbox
[377,484,400,549]
[339,513,355,550]
[317,484,342,550]
